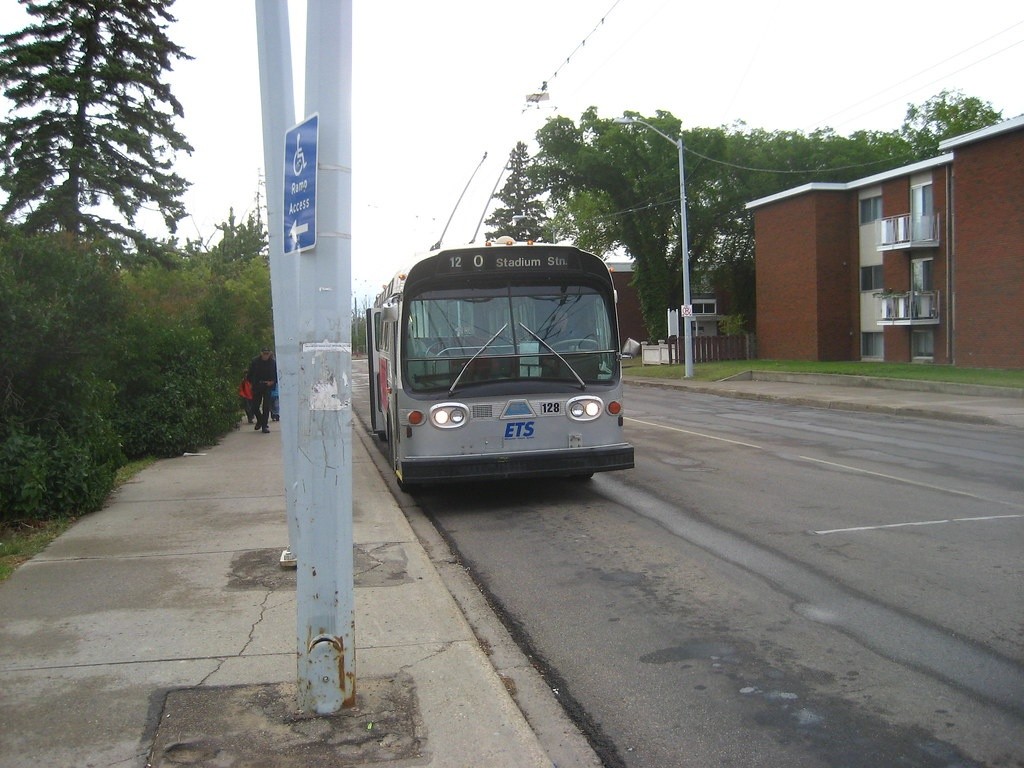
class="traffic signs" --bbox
[281,111,317,255]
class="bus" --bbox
[364,153,638,494]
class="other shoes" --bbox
[273,414,279,421]
[262,427,270,432]
[255,422,262,429]
[248,419,254,424]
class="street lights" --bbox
[612,117,693,379]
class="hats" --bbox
[260,346,270,354]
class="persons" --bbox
[270,352,279,421]
[540,312,585,364]
[239,371,254,424]
[247,347,276,432]
